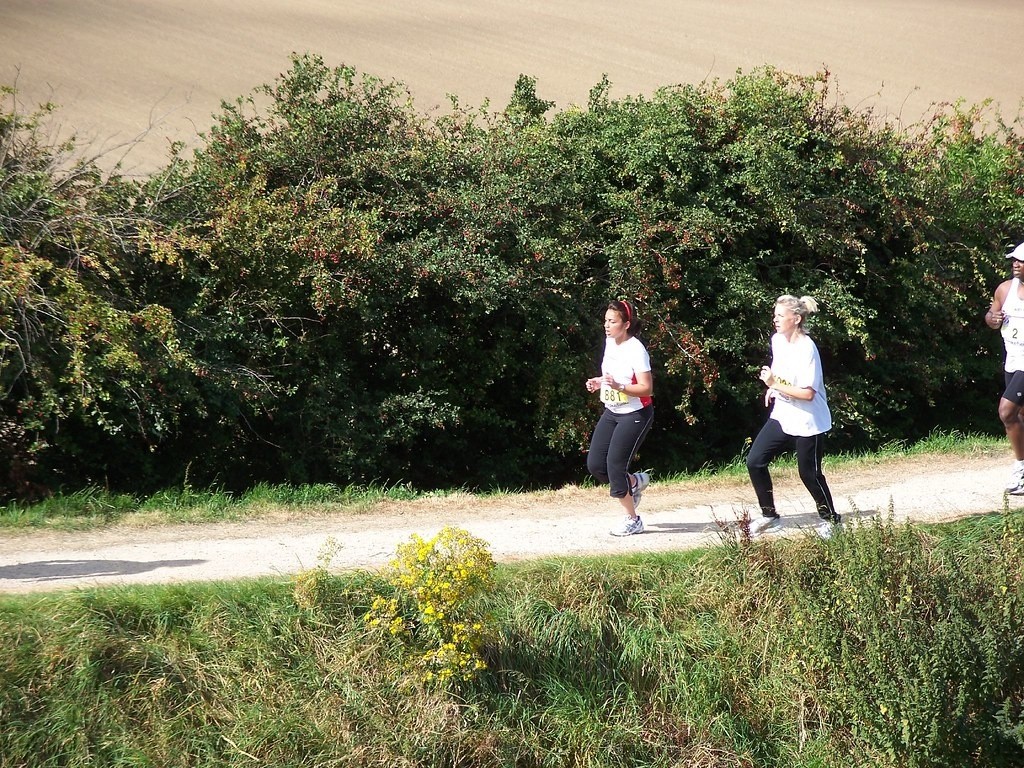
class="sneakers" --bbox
[1005,471,1024,495]
[820,515,844,536]
[744,515,782,537]
[632,473,650,509]
[610,516,645,536]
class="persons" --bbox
[586,300,654,537]
[985,242,1024,495]
[739,295,842,538]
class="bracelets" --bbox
[619,384,625,392]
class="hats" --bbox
[1006,243,1024,261]
